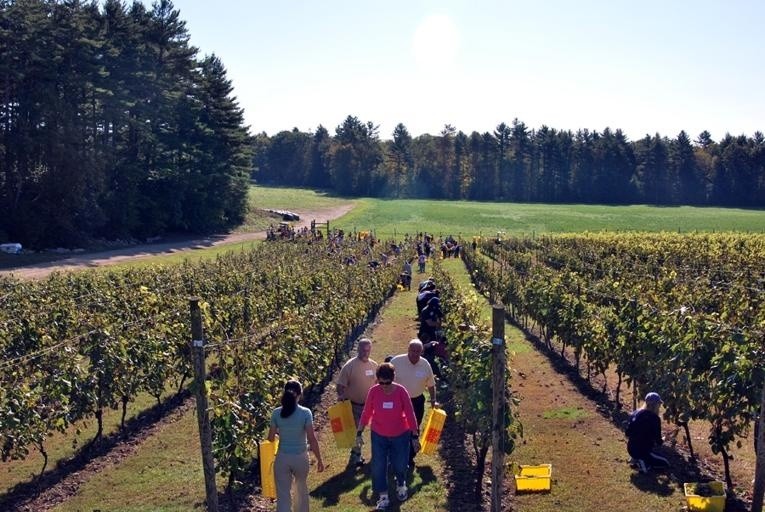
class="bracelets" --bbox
[356,431,363,436]
[412,434,419,440]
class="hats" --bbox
[645,392,664,405]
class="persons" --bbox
[336,337,380,473]
[624,392,673,475]
[389,338,441,472]
[355,362,421,512]
[265,220,507,381]
[268,382,324,512]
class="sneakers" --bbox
[376,498,391,512]
[636,459,647,473]
[350,451,365,464]
[396,480,408,501]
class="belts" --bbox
[350,401,364,406]
